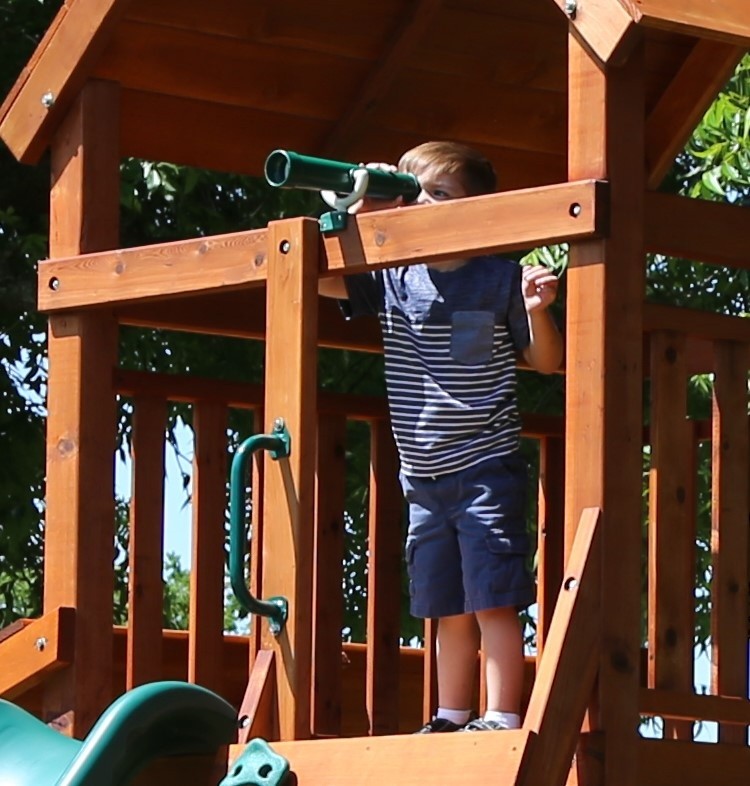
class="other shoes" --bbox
[412,715,463,735]
[457,716,507,731]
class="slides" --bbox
[0,679,238,786]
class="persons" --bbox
[345,138,563,734]
